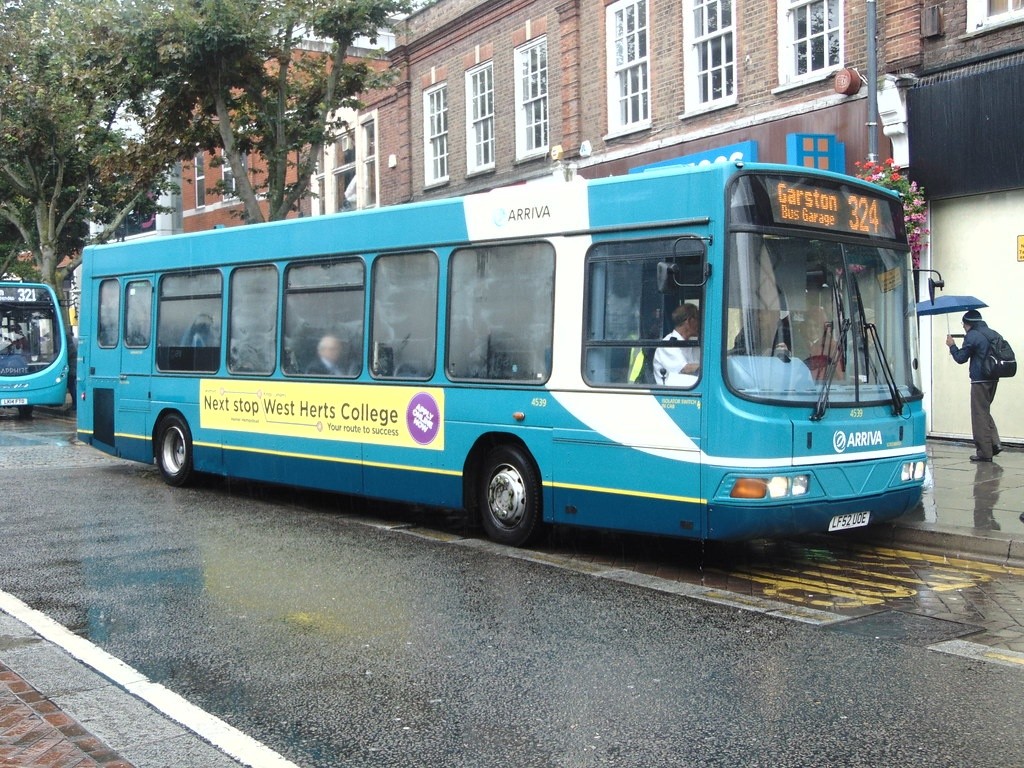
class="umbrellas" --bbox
[916,295,989,335]
[40,337,46,342]
[43,332,50,337]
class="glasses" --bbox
[960,322,965,325]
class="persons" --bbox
[651,304,701,386]
[945,310,1003,462]
[304,336,344,376]
[802,307,844,381]
[66,334,77,412]
[732,311,796,356]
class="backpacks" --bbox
[978,327,1018,378]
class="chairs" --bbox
[181,325,423,376]
[625,327,656,383]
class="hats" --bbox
[962,311,982,325]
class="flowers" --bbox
[809,158,933,277]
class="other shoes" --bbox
[970,455,992,462]
[992,443,1003,455]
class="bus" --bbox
[0,281,78,418]
[76,161,943,549]
